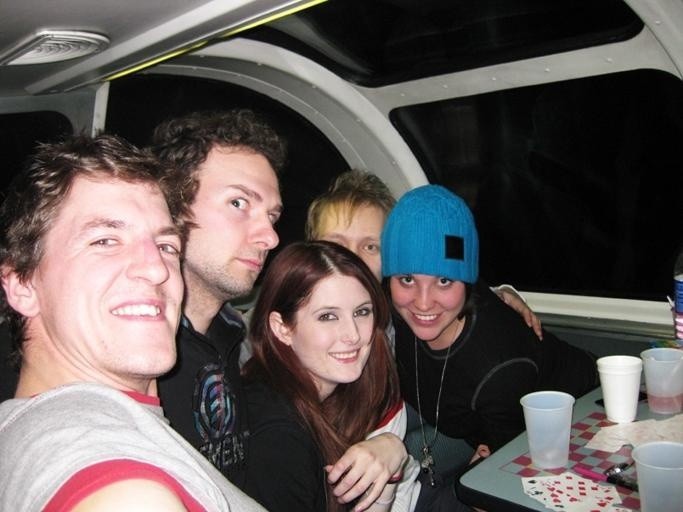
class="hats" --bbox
[379,185,482,285]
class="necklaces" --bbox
[410,319,462,489]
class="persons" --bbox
[238,236,404,510]
[0,121,273,512]
[235,167,545,374]
[154,102,284,492]
[375,182,602,468]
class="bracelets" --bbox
[363,489,395,505]
[386,469,405,486]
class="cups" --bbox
[519,389,576,471]
[630,440,683,512]
[595,355,643,424]
[639,347,683,414]
